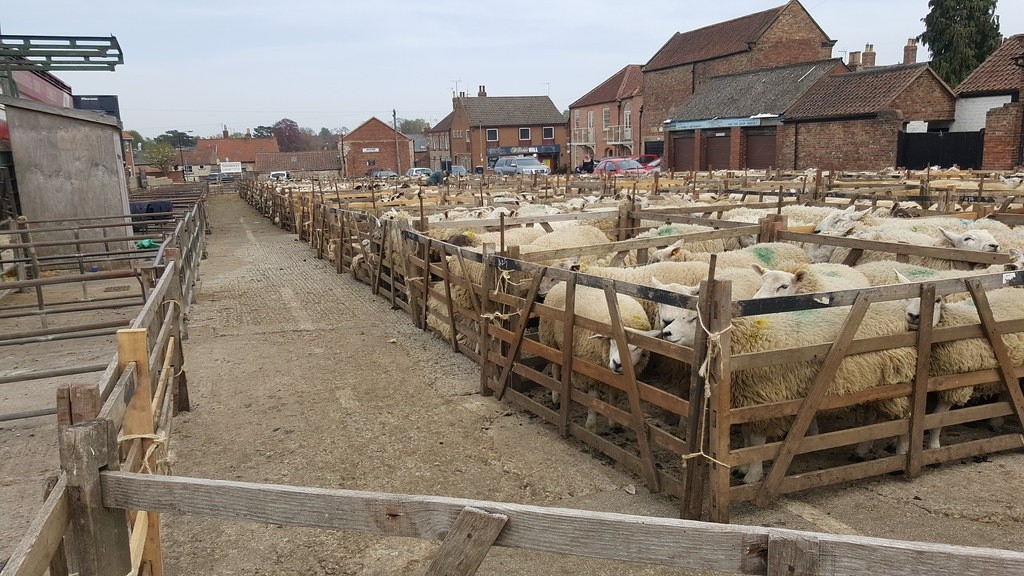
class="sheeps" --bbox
[257,165,1023,484]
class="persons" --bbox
[580,155,593,173]
[427,169,450,186]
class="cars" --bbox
[644,157,662,172]
[207,172,235,183]
[405,167,433,179]
[270,170,286,179]
[628,154,658,167]
[593,157,647,175]
[368,168,397,178]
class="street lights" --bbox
[178,130,194,182]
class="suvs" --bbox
[450,164,471,175]
[494,156,552,176]
